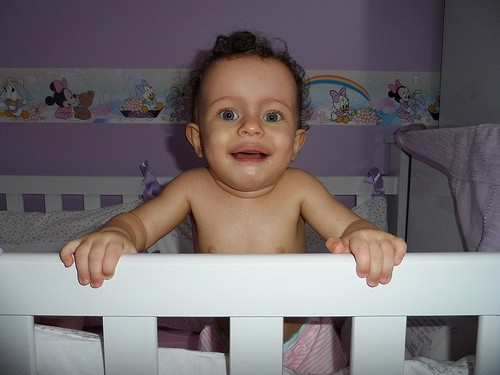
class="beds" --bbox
[0,120,500,375]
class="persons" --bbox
[61,28,407,374]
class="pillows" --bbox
[404,314,478,363]
